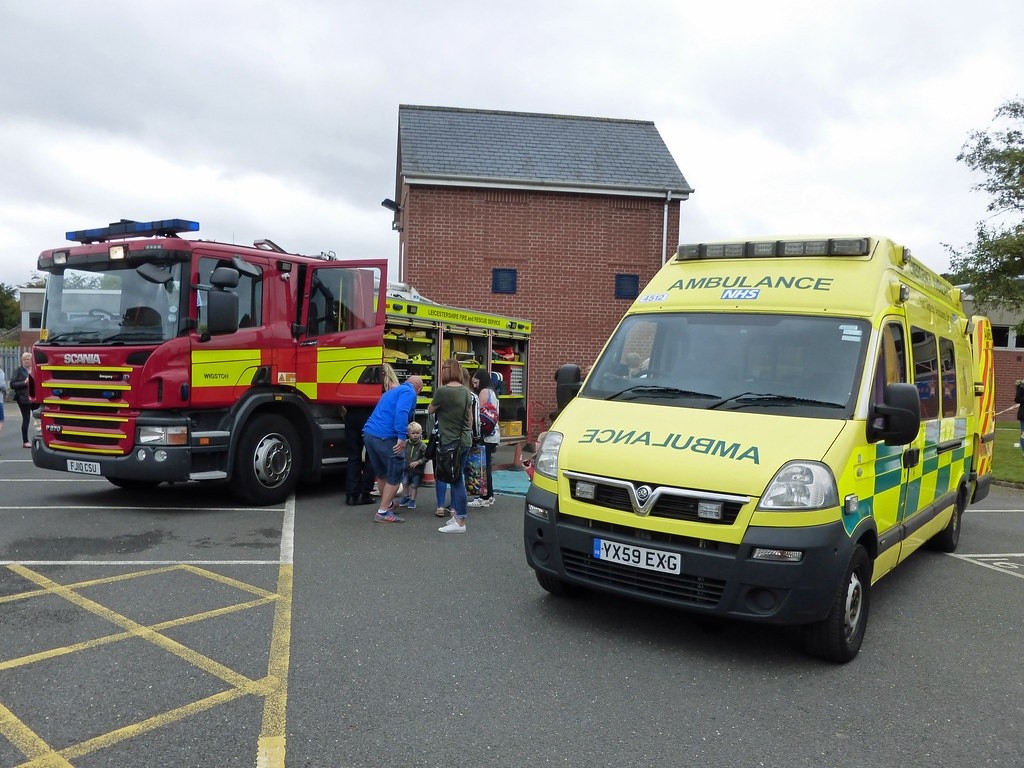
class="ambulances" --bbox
[524,233,995,667]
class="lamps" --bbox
[381,199,400,212]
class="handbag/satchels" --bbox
[480,389,498,435]
[434,445,461,484]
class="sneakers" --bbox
[445,505,455,512]
[386,501,399,510]
[467,497,495,508]
[399,495,416,509]
[436,507,444,516]
[438,516,466,533]
[374,510,405,523]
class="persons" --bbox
[1014,379,1024,453]
[10,352,39,448]
[340,363,401,506]
[423,367,481,516]
[467,368,500,507]
[521,431,548,481]
[0,369,7,422]
[583,353,650,381]
[427,359,472,533]
[399,422,429,509]
[362,376,423,524]
[549,411,561,426]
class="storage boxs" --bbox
[497,421,522,436]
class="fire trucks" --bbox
[28,219,532,508]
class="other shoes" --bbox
[345,497,357,506]
[360,496,377,505]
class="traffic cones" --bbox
[509,441,527,471]
